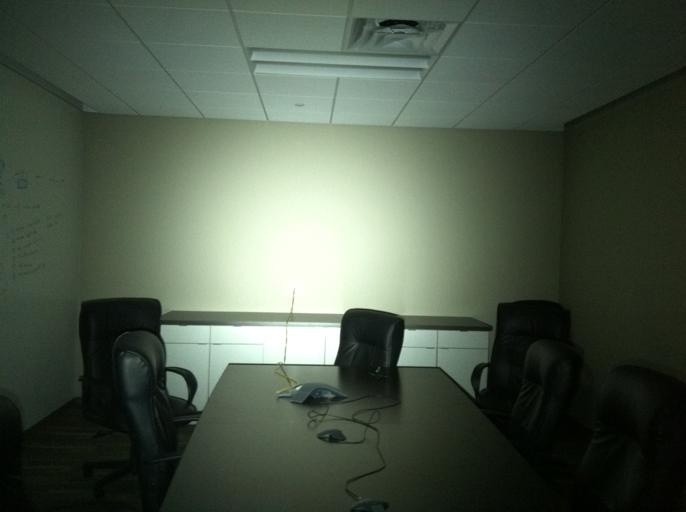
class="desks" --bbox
[155,359,579,511]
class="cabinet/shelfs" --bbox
[160,307,494,415]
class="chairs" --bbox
[76,297,166,496]
[112,330,202,511]
[509,339,586,475]
[334,306,409,370]
[470,299,577,430]
[565,362,684,510]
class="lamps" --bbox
[248,48,430,81]
